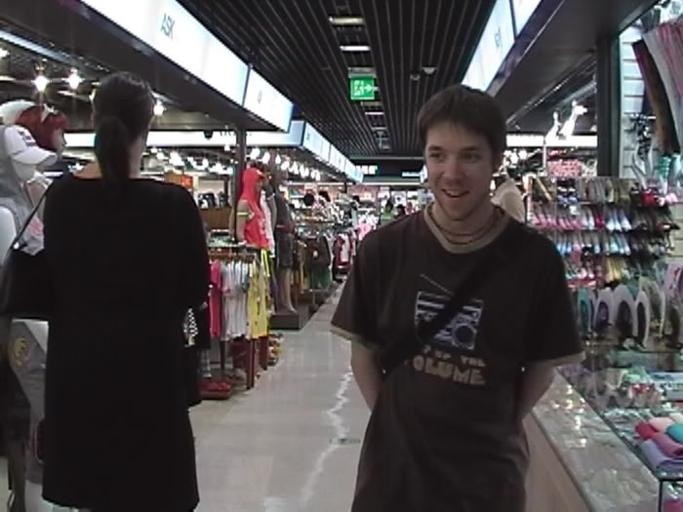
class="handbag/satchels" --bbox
[0,175,60,320]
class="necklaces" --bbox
[428,204,496,237]
[426,201,505,246]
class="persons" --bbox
[0,123,82,511]
[394,204,407,221]
[297,191,328,218]
[15,104,71,225]
[327,83,588,512]
[229,158,298,317]
[39,70,213,512]
[378,202,395,227]
